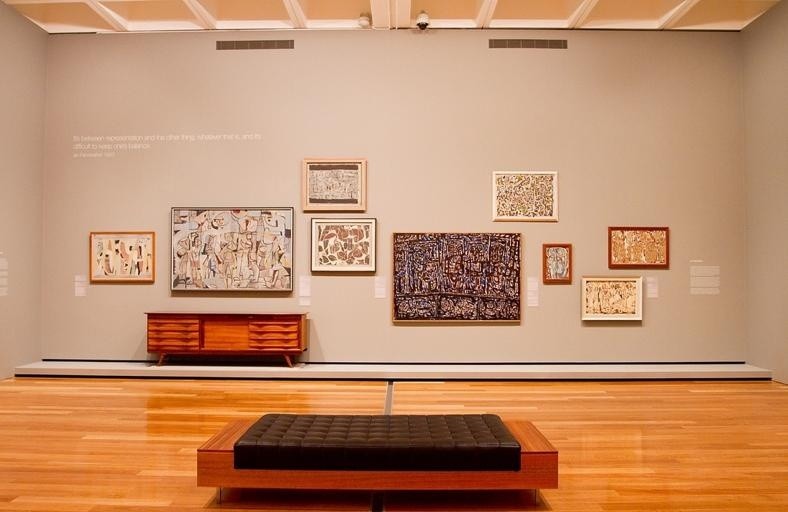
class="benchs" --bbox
[194,411,560,511]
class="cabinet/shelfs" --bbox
[140,311,310,368]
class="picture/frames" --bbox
[170,206,294,294]
[607,226,670,270]
[391,232,521,324]
[542,243,572,285]
[310,218,377,275]
[90,232,155,285]
[581,277,643,321]
[492,171,559,222]
[301,158,368,212]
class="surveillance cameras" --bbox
[417,13,429,30]
